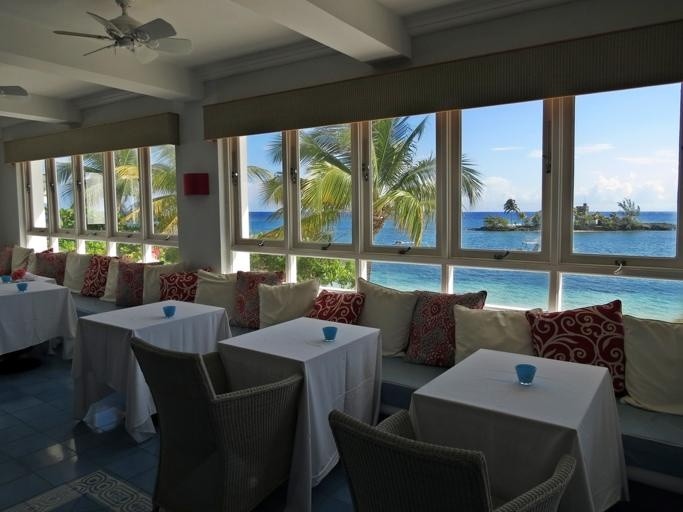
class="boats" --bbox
[392,240,414,247]
[511,241,541,251]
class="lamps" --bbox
[132,45,159,65]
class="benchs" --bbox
[229,322,256,341]
[380,356,446,416]
[616,399,683,493]
[71,294,132,319]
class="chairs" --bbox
[328,408,577,512]
[130,336,305,512]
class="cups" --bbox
[322,326,337,341]
[517,365,536,385]
[162,306,175,317]
[2,274,11,282]
[17,283,27,291]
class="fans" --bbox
[50,0,194,66]
[0,85,29,96]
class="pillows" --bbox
[193,268,238,315]
[11,245,34,274]
[229,270,284,328]
[453,303,543,366]
[303,288,366,325]
[257,278,321,331]
[115,260,164,306]
[97,257,121,303]
[158,264,212,303]
[26,248,53,274]
[79,253,121,297]
[403,289,488,367]
[524,299,626,400]
[619,314,683,417]
[0,244,18,275]
[142,262,185,306]
[62,252,93,294]
[350,277,419,359]
[35,252,67,285]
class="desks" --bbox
[72,299,232,447]
[0,281,79,362]
[408,348,629,512]
[216,317,383,488]
